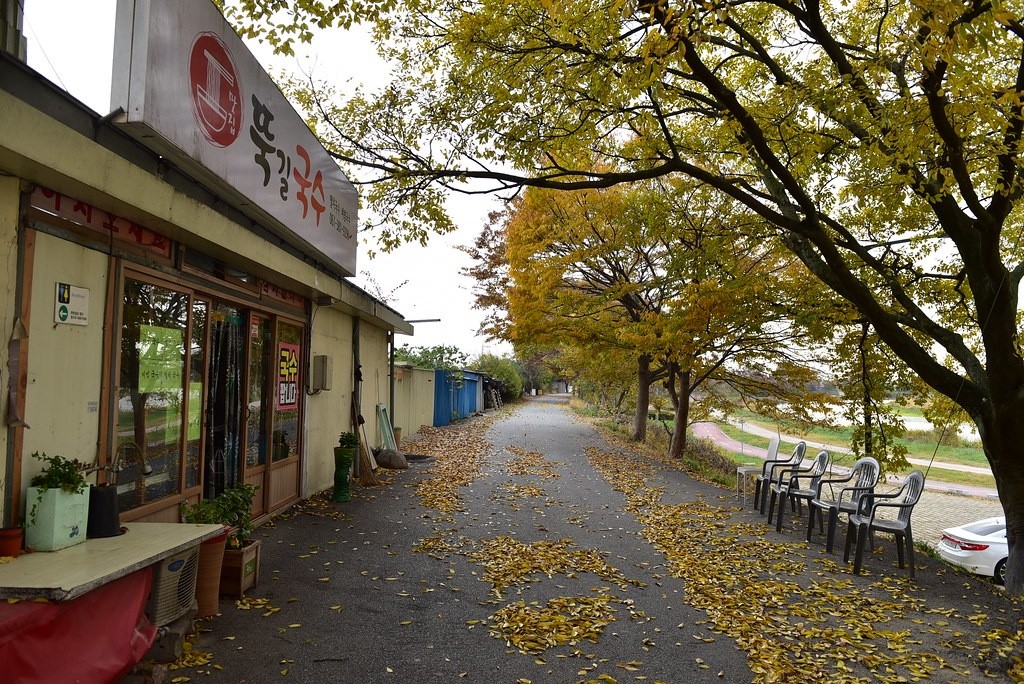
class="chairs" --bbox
[807,457,880,553]
[736,438,779,507]
[754,441,806,517]
[768,449,830,533]
[843,470,925,578]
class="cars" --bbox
[937,516,1009,585]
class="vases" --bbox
[0,528,24,558]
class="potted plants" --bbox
[335,431,358,502]
[178,498,231,618]
[215,481,263,600]
[17,451,90,551]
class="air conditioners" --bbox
[148,544,200,626]
